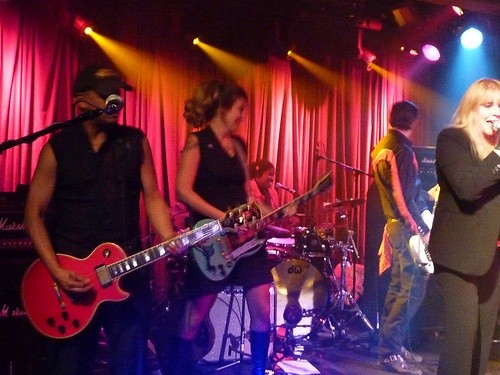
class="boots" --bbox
[173,339,193,375]
[248,325,271,375]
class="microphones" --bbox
[314,143,320,161]
[275,182,296,193]
[493,120,500,132]
[105,93,124,114]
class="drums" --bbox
[265,237,297,257]
[264,253,331,342]
[292,225,331,257]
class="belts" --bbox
[387,218,401,221]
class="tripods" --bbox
[313,204,375,340]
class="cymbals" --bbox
[323,198,365,211]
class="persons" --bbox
[426,78,500,375]
[370,101,448,375]
[242,158,280,210]
[24,63,197,375]
[170,80,300,375]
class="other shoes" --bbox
[400,345,423,363]
[380,354,422,375]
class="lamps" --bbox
[416,34,448,65]
[191,32,201,45]
[71,15,95,41]
[450,12,488,51]
[353,47,376,73]
[279,46,294,57]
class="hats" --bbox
[74,63,135,93]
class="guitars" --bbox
[22,199,263,339]
[408,186,440,274]
[191,169,333,283]
